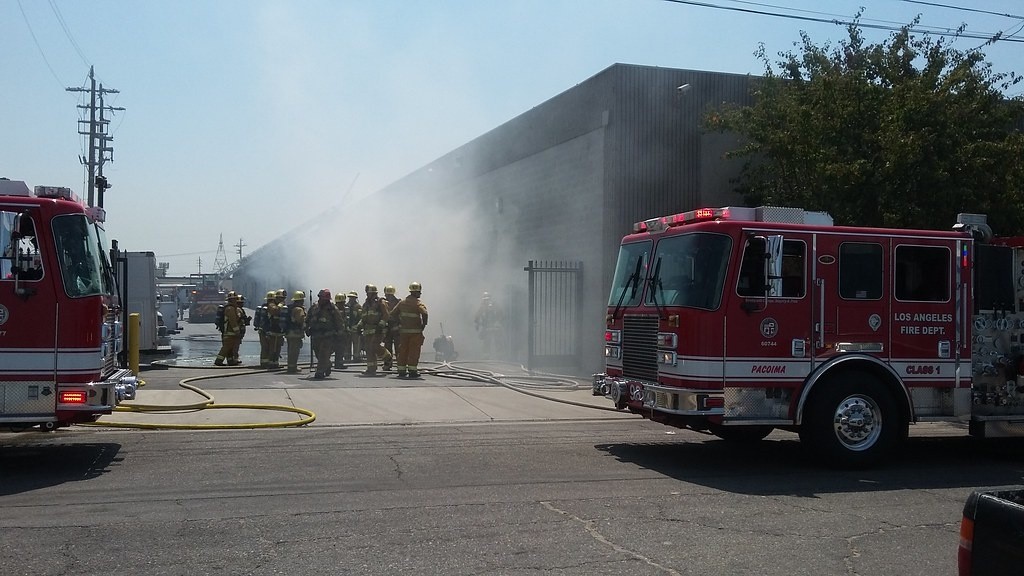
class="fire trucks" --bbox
[187,273,229,323]
[0,61,150,431]
[590,207,1024,474]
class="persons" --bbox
[233,294,252,363]
[212,291,242,366]
[474,291,507,360]
[255,282,429,380]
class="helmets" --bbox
[482,292,491,299]
[226,282,422,303]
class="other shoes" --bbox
[214,356,422,379]
